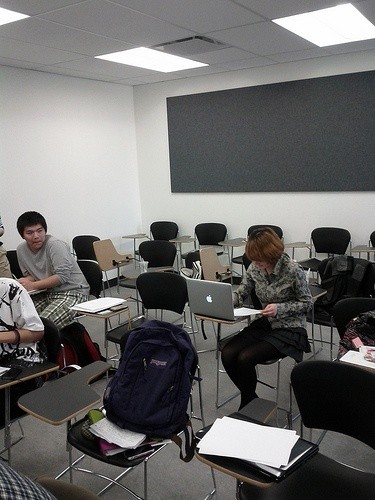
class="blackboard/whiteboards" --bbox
[166,70,375,192]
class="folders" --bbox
[98,438,157,457]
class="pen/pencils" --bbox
[12,274,17,280]
[0,370,8,375]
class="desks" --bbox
[0,234,375,488]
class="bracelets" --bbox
[11,329,20,344]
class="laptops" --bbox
[185,278,252,321]
[197,411,320,483]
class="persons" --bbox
[0,216,12,278]
[0,277,45,372]
[13,211,90,331]
[221,227,315,412]
[0,456,93,500]
[338,311,375,363]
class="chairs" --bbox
[5,220,375,500]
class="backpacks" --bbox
[102,320,199,463]
[59,321,118,385]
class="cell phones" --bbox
[2,367,21,380]
[110,305,127,311]
[124,443,154,460]
